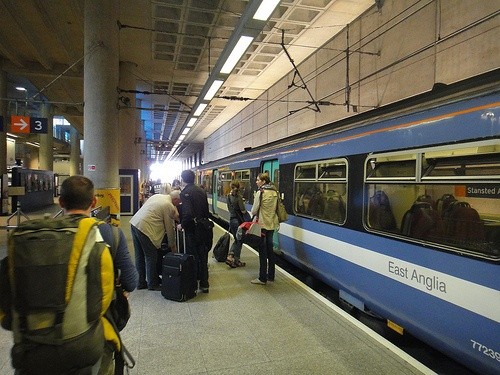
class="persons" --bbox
[0,175,138,375]
[251,173,281,285]
[225,181,251,268]
[130,169,215,294]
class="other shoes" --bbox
[250,278,266,285]
[195,287,210,294]
[226,258,237,268]
[235,261,245,267]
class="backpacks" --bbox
[6,213,136,375]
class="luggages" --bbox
[213,227,231,262]
[161,227,197,302]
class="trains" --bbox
[176,86,500,372]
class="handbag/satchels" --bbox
[110,225,131,331]
[194,217,215,255]
[249,215,262,238]
[239,211,251,222]
[235,215,265,241]
[276,191,288,223]
